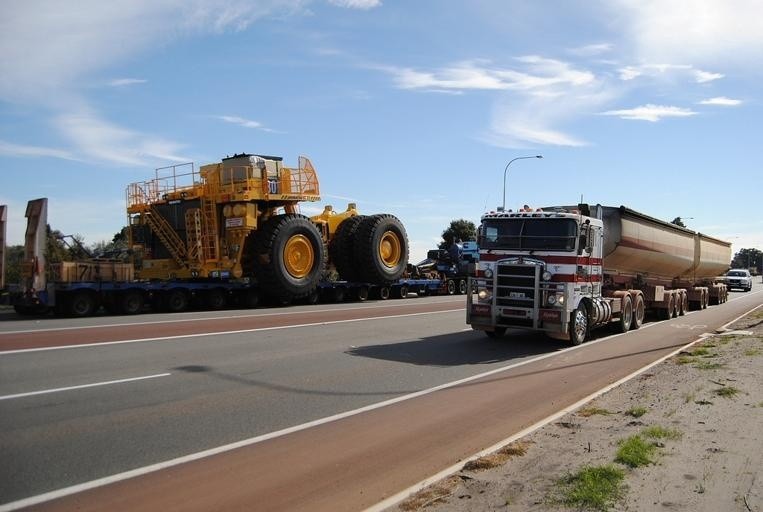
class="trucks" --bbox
[0,198,478,319]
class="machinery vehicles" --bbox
[124,152,409,297]
[465,198,731,345]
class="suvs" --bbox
[725,269,752,292]
